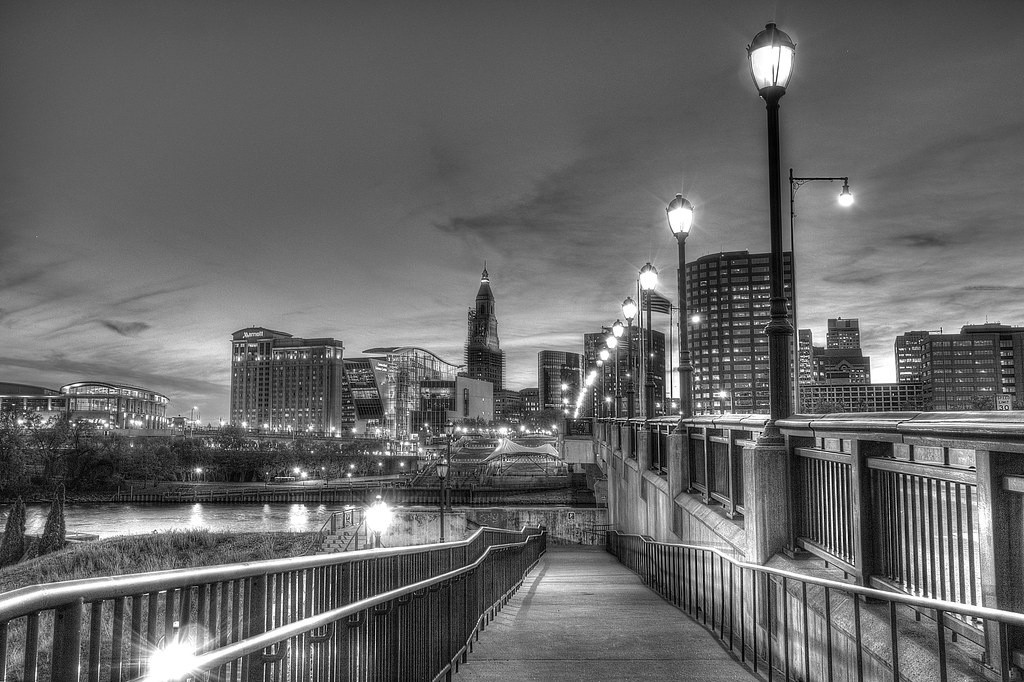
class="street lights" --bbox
[620,296,638,415]
[435,455,450,543]
[639,261,659,416]
[573,320,626,421]
[747,22,798,422]
[191,407,198,439]
[788,169,854,422]
[666,195,696,419]
